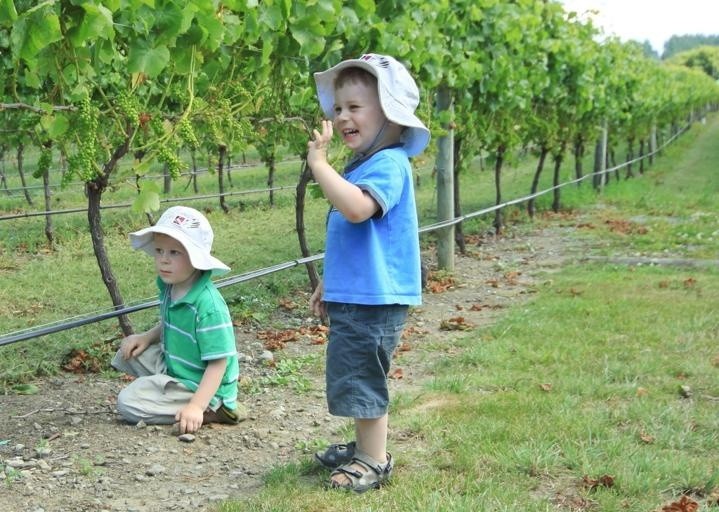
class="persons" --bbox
[303,51,431,492]
[110,205,241,439]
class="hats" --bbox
[126,208,233,278]
[311,52,431,162]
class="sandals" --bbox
[313,440,356,467]
[328,451,396,492]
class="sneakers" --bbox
[216,401,247,424]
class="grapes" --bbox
[20,80,575,194]
